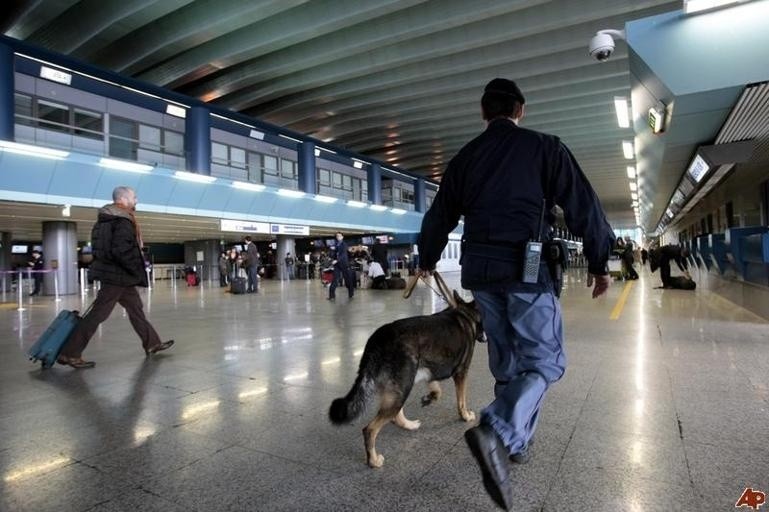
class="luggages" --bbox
[231,261,247,294]
[28,296,98,369]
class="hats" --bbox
[484,78,525,105]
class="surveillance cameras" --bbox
[588,34,615,63]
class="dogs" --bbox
[328,289,490,469]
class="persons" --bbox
[326,231,354,300]
[415,76,616,512]
[29,250,44,296]
[649,245,692,289]
[218,236,389,293]
[406,248,416,276]
[53,185,176,370]
[617,235,647,280]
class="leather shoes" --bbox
[464,415,513,511]
[57,350,96,369]
[146,340,174,357]
